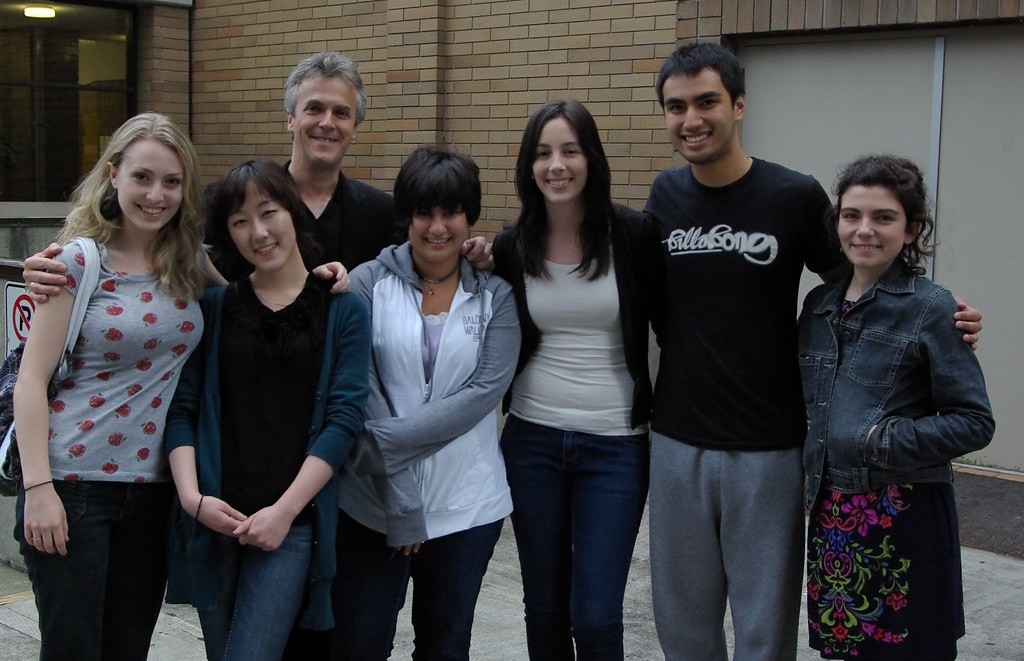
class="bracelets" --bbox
[195,494,204,519]
[25,481,53,491]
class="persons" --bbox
[488,99,654,660]
[163,158,372,661]
[14,110,351,661]
[331,146,522,660]
[797,155,996,661]
[643,40,984,661]
[21,49,496,660]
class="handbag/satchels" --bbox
[0,235,100,494]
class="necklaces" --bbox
[414,264,460,294]
